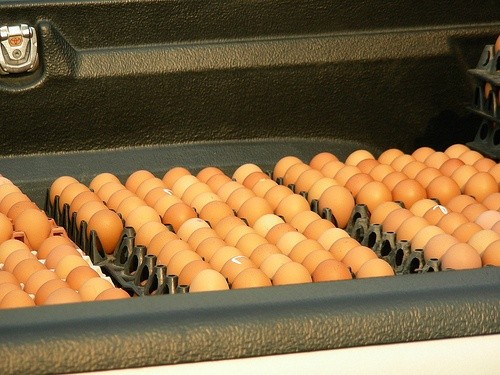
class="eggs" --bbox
[49,163,395,292]
[273,144,500,271]
[0,174,132,308]
